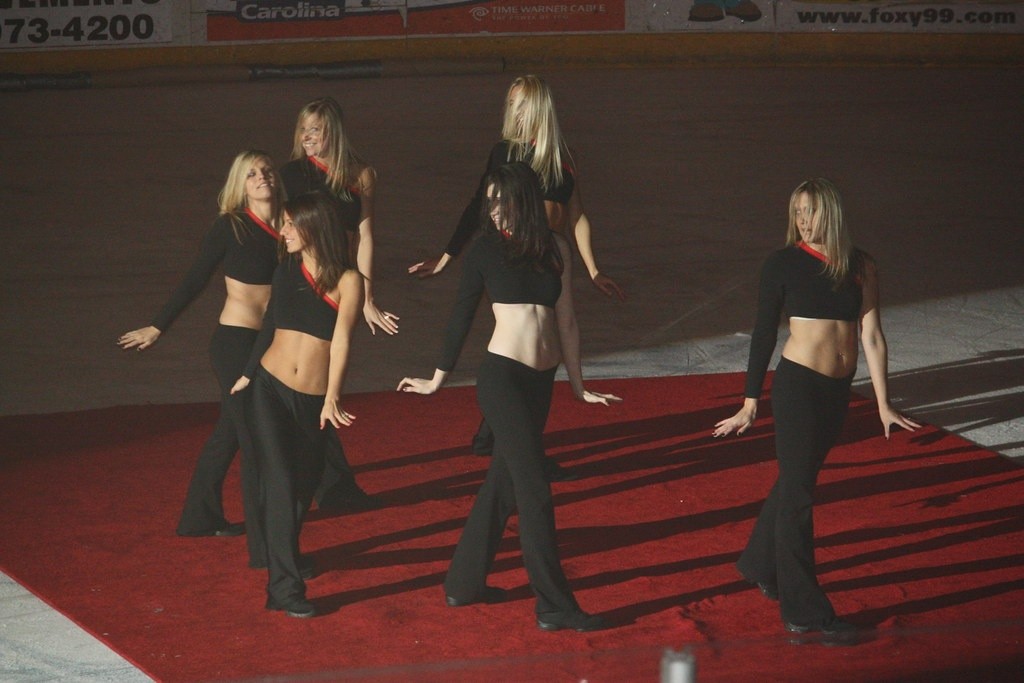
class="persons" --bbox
[115,96,400,616]
[711,176,924,632]
[396,163,622,631]
[405,75,620,482]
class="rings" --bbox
[385,316,389,319]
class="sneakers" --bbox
[784,618,859,647]
[738,567,780,600]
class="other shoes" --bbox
[215,524,244,536]
[249,557,315,578]
[266,598,316,618]
[357,495,379,511]
[445,587,508,607]
[544,462,579,482]
[537,610,609,632]
